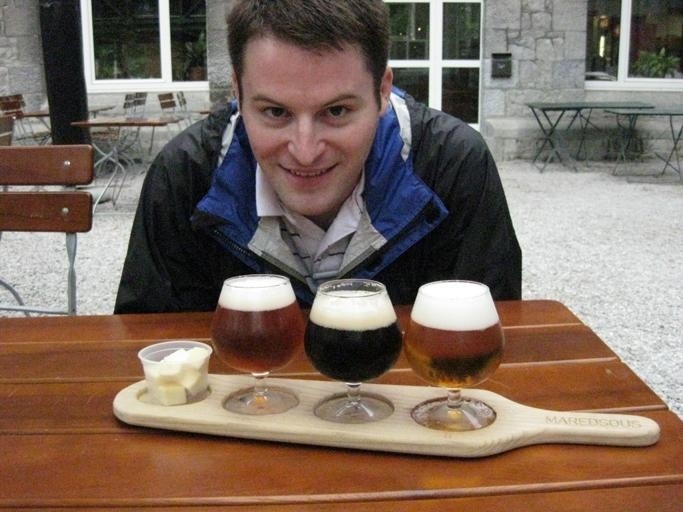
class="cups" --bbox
[138,340,212,405]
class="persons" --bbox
[113,0,522,314]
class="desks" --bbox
[604,107,683,179]
[524,98,655,173]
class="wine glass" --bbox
[208,273,305,414]
[404,279,504,434]
[301,277,404,426]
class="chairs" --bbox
[0,90,221,218]
[0,143,99,317]
